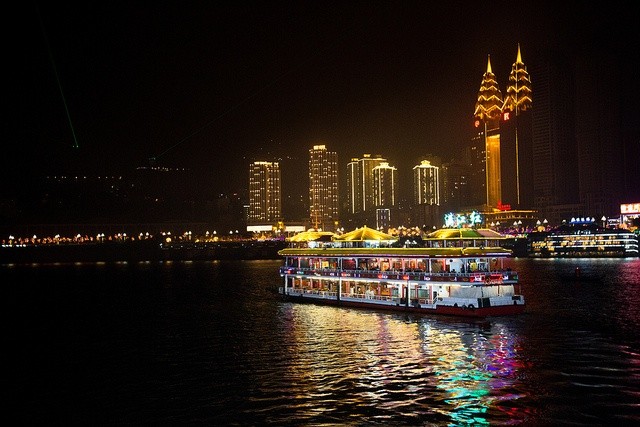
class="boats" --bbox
[277,225,526,317]
